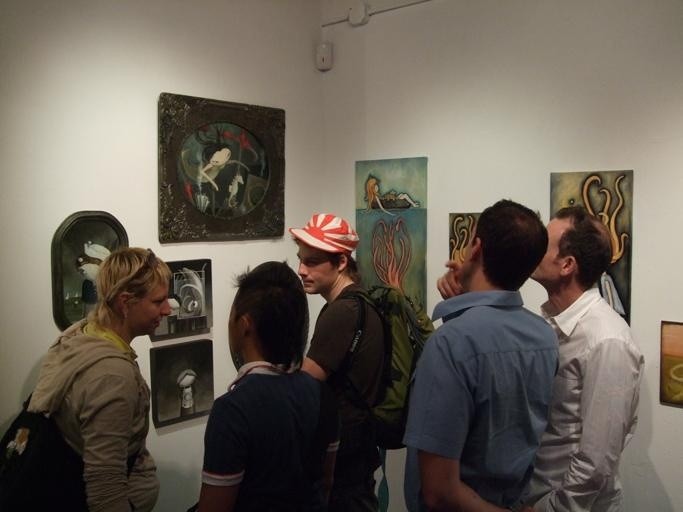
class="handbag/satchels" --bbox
[0,391,136,512]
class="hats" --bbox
[289,212,360,256]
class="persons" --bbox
[185,261,341,512]
[530,208,645,512]
[401,198,560,512]
[0,247,172,512]
[288,213,384,512]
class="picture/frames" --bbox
[144,257,216,430]
[156,91,287,248]
[50,210,131,333]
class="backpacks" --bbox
[328,284,434,449]
